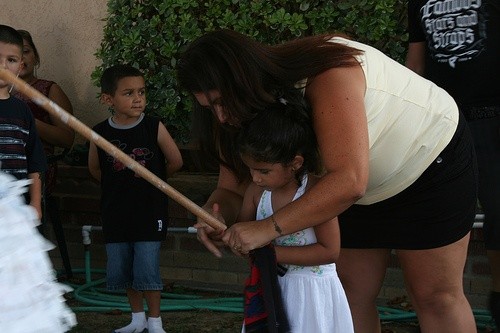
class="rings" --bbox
[234,248,242,251]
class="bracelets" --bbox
[271,215,284,237]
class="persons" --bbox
[177,29,479,333]
[403,0,500,327]
[88,65,183,333]
[193,99,355,333]
[0,25,76,333]
